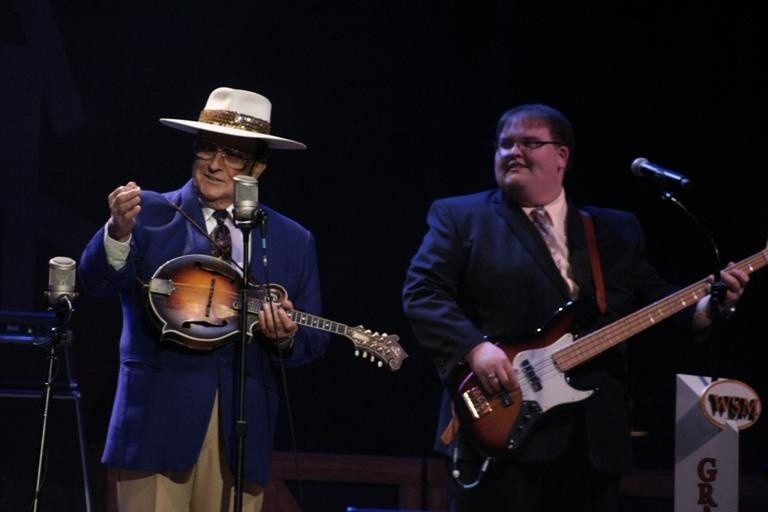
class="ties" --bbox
[531,207,561,276]
[209,208,232,261]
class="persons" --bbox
[400,102,749,511]
[77,88,328,511]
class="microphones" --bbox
[632,157,690,190]
[45,256,79,329]
[231,175,269,234]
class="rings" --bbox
[487,373,495,379]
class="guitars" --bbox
[147,253,409,373]
[448,243,767,456]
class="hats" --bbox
[161,87,306,150]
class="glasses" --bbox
[494,137,559,150]
[191,138,250,171]
